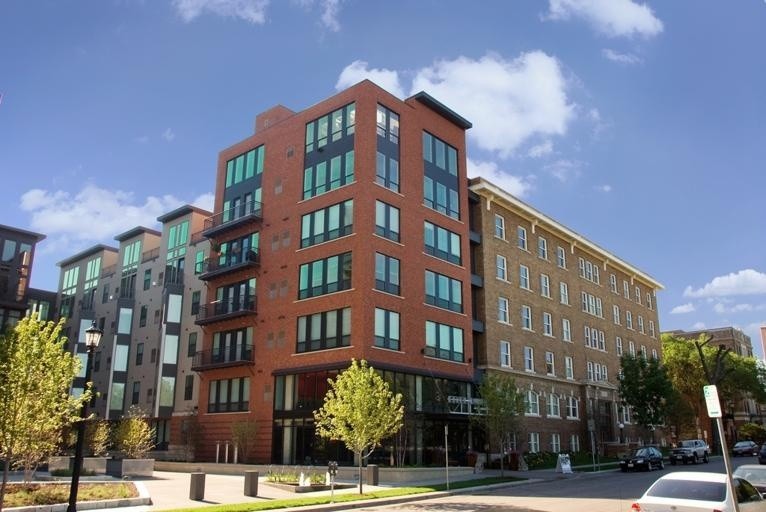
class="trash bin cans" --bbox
[190,473,205,500]
[244,470,259,496]
[367,465,378,486]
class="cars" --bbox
[620,439,710,471]
[632,465,766,512]
[731,440,766,465]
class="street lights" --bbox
[67,320,103,512]
